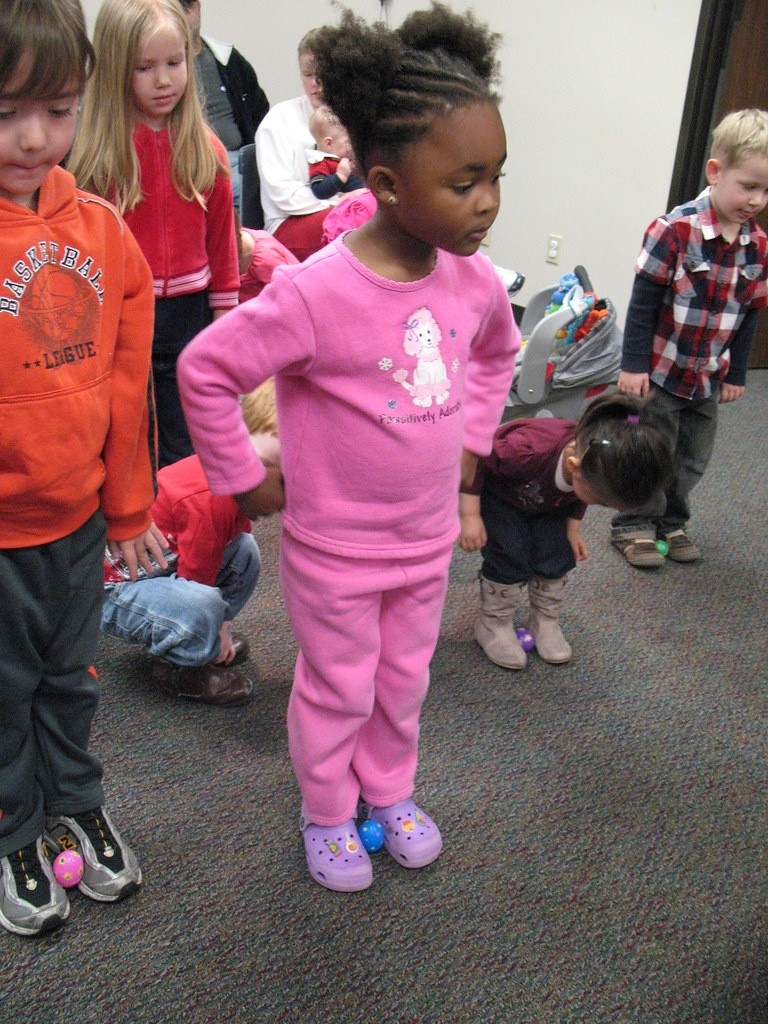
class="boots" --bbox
[473,569,528,669]
[529,574,572,664]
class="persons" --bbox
[610,108,768,566]
[460,389,679,669]
[65,0,241,473]
[0,0,171,939]
[100,0,380,699]
[176,0,522,896]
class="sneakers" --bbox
[0,834,72,935]
[663,530,702,561]
[614,538,665,566]
[43,805,143,901]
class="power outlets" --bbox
[544,233,564,266]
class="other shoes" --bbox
[359,797,443,868]
[300,803,373,891]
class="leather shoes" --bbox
[230,634,249,665]
[152,662,252,706]
